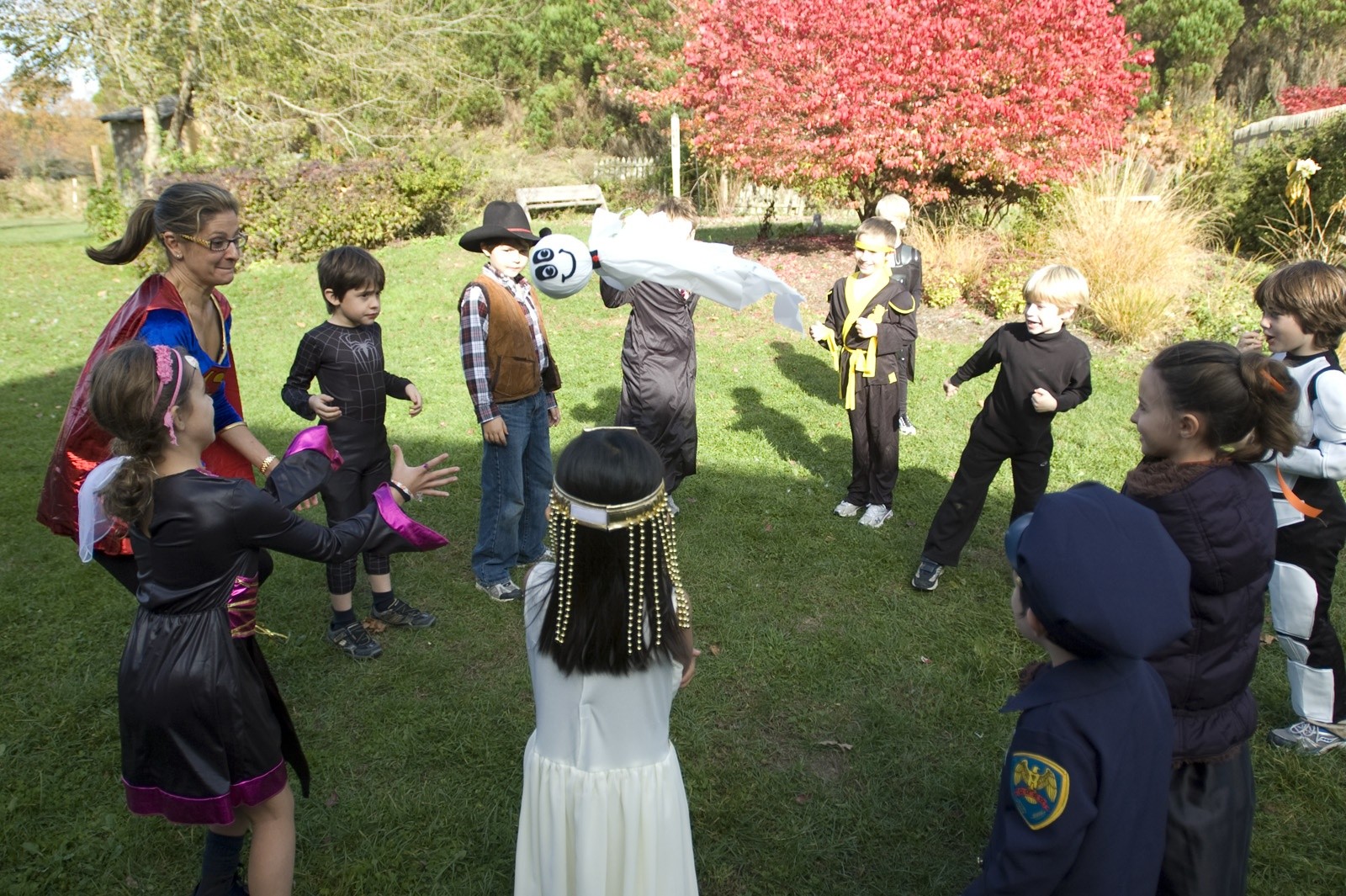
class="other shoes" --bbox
[192,864,248,896]
[667,495,680,515]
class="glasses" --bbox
[160,232,248,251]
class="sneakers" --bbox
[327,620,382,661]
[834,500,869,517]
[858,503,893,528]
[517,547,553,568]
[898,415,916,434]
[1267,722,1346,756]
[475,579,524,602]
[912,558,944,590]
[372,598,436,628]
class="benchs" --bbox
[515,184,609,222]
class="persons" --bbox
[810,191,923,528]
[616,194,704,516]
[455,200,564,605]
[912,263,1093,591]
[512,425,704,896]
[1118,339,1303,896]
[968,480,1192,896]
[78,338,463,896]
[1233,259,1346,759]
[35,180,319,597]
[281,246,438,664]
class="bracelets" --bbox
[389,479,425,504]
[260,453,277,475]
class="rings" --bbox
[422,462,431,471]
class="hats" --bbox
[1004,481,1193,663]
[458,201,541,253]
